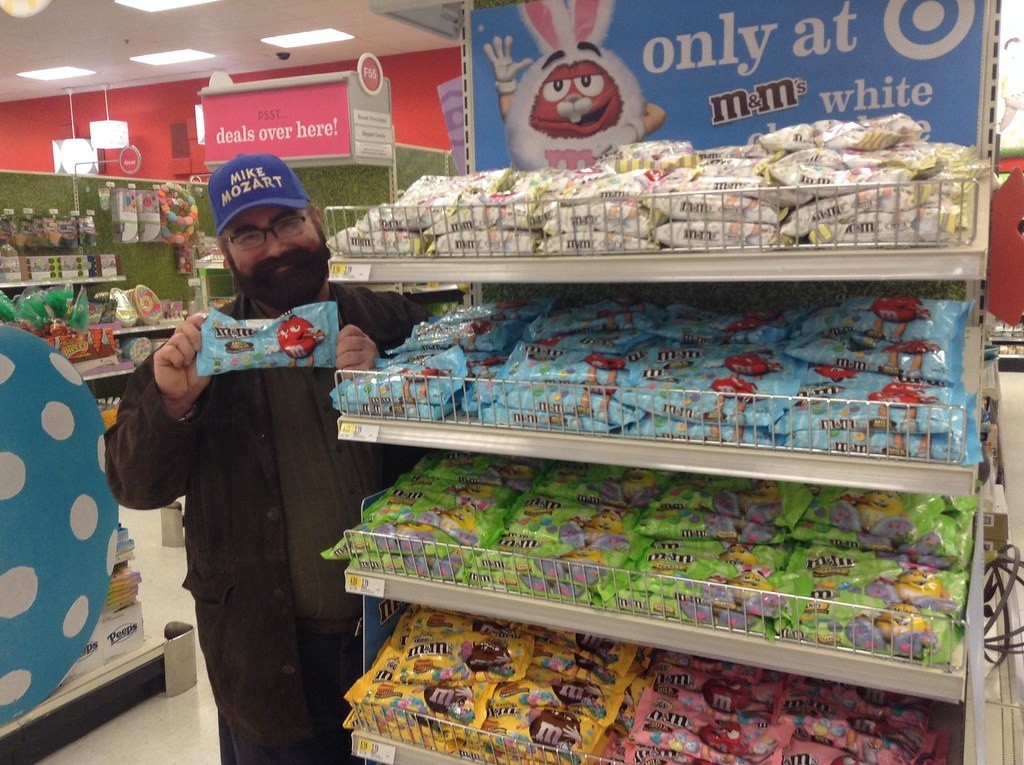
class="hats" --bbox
[208,153,311,236]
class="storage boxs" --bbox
[985,356,1010,555]
[15,322,115,362]
[1,255,122,283]
[176,243,193,275]
[103,598,143,659]
[62,615,104,685]
[99,403,118,429]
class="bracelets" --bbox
[176,405,197,420]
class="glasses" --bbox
[222,212,309,251]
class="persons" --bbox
[99,154,429,764]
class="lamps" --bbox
[195,104,206,146]
[51,87,107,175]
[89,83,129,150]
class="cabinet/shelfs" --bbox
[323,151,985,765]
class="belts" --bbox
[295,617,363,636]
